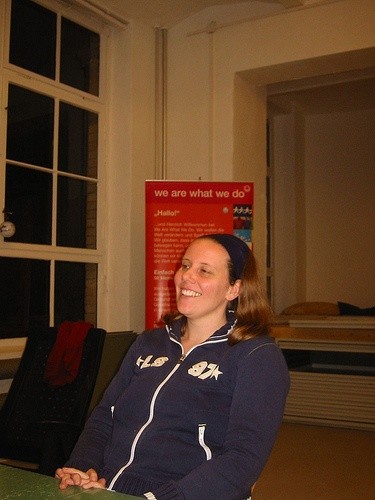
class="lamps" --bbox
[0,206,16,239]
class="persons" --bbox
[54,233,290,500]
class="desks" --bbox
[0,464,149,500]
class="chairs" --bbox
[0,327,139,478]
